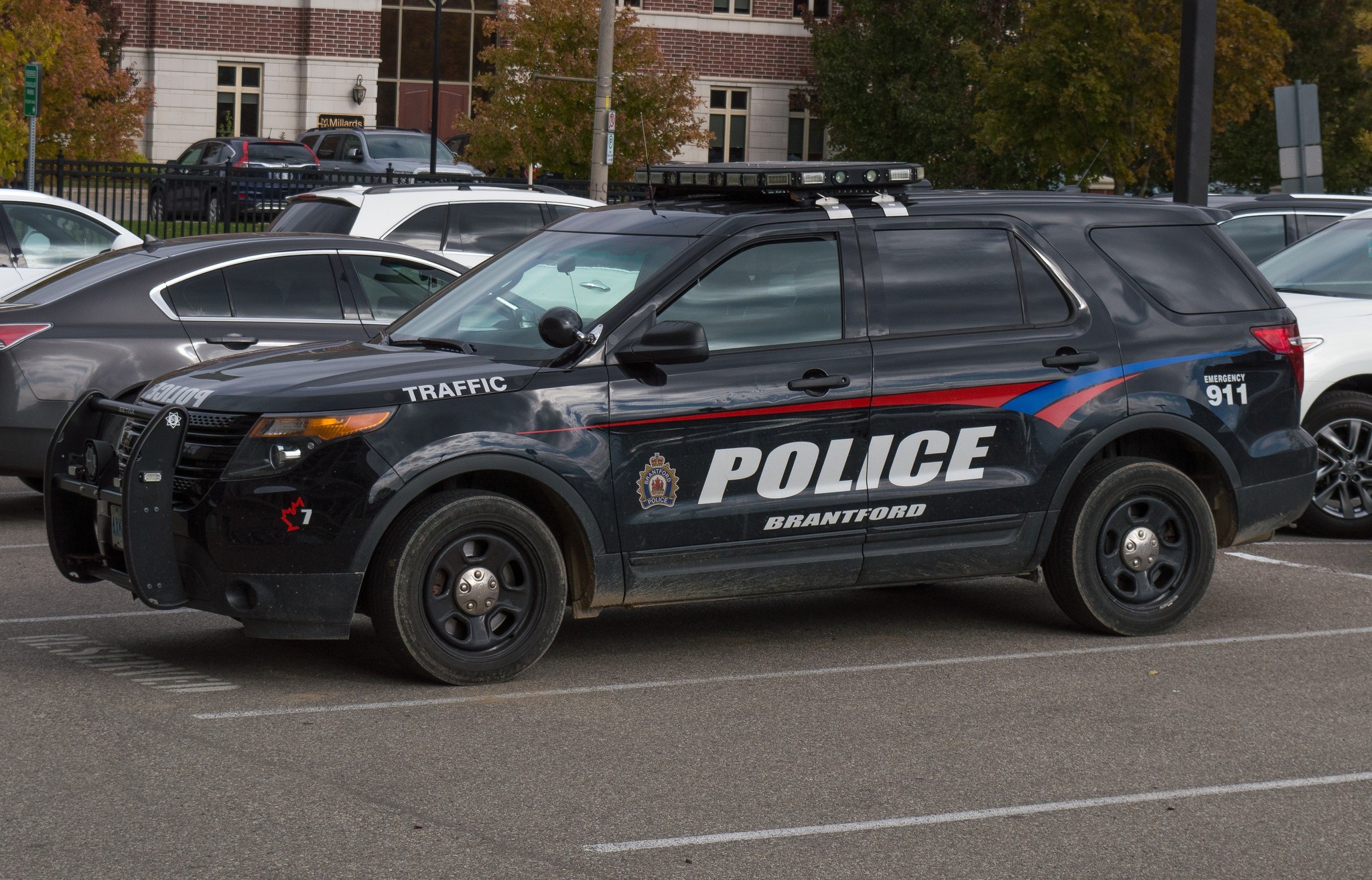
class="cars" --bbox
[1,233,551,500]
[298,129,487,188]
[148,138,323,225]
[1,188,145,300]
[261,180,643,317]
[1155,192,1372,539]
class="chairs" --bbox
[376,296,416,322]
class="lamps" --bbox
[353,75,367,106]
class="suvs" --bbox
[39,159,1318,694]
